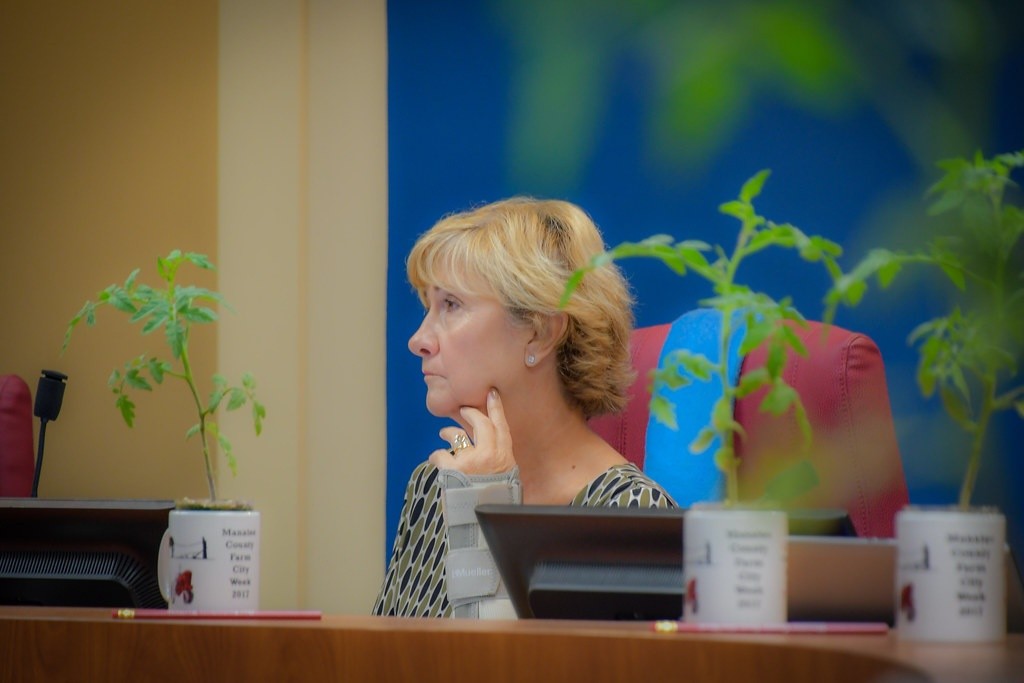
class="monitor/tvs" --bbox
[0,498,176,610]
[476,504,858,626]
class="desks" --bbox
[0,607,1024,683]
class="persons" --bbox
[371,197,680,619]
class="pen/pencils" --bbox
[108,608,323,623]
[649,619,890,635]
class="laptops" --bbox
[784,534,1010,637]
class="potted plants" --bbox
[824,147,1024,641]
[558,167,869,630]
[58,248,266,613]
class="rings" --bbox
[454,434,471,452]
